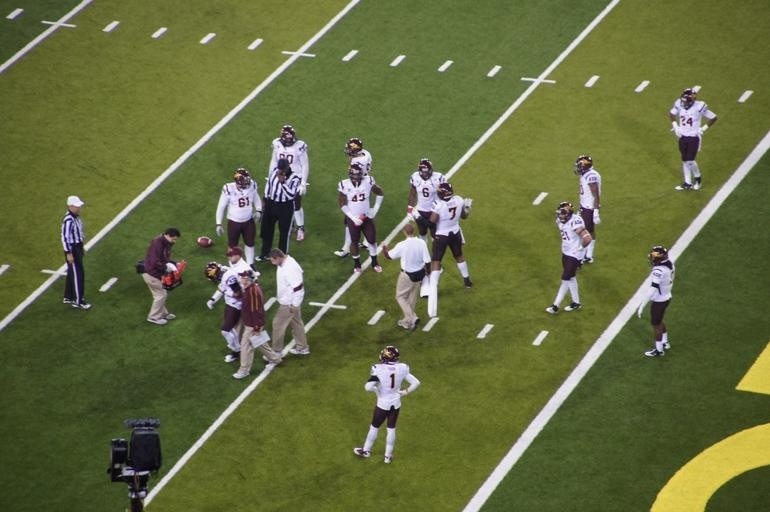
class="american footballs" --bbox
[196,236,215,247]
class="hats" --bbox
[237,269,254,277]
[65,195,85,207]
[273,159,291,175]
[225,247,243,256]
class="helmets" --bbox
[555,201,574,224]
[680,87,697,110]
[348,162,364,182]
[344,138,363,156]
[573,155,593,176]
[281,125,295,146]
[379,346,401,365]
[437,183,454,202]
[647,246,669,266]
[204,262,220,281]
[417,158,433,180]
[233,168,251,186]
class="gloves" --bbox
[464,197,473,208]
[593,209,601,224]
[216,225,225,237]
[368,195,384,219]
[339,205,364,227]
[167,263,177,272]
[253,211,263,224]
[699,124,709,135]
[297,183,307,197]
[577,243,585,251]
[406,205,416,223]
[398,389,408,398]
[637,301,647,319]
[672,121,683,139]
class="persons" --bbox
[545,201,592,314]
[668,87,718,191]
[637,245,676,357]
[353,346,421,464]
[383,158,472,331]
[59,195,92,310]
[215,125,309,272]
[141,228,181,325]
[576,155,602,264]
[205,246,310,378]
[333,139,384,272]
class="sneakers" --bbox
[165,313,177,319]
[146,318,169,325]
[288,347,311,356]
[654,342,672,349]
[353,447,371,458]
[333,248,351,258]
[262,349,287,361]
[254,255,271,262]
[72,300,92,310]
[693,177,702,190]
[675,183,693,191]
[354,265,364,273]
[409,317,420,331]
[396,319,409,330]
[383,453,394,464]
[232,369,251,379]
[63,297,76,304]
[265,360,283,370]
[545,304,559,314]
[564,303,582,312]
[296,225,305,241]
[371,261,383,273]
[224,353,239,363]
[643,347,666,357]
[250,264,262,277]
[358,242,369,248]
[464,276,473,289]
[581,257,597,263]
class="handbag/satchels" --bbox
[407,269,426,283]
